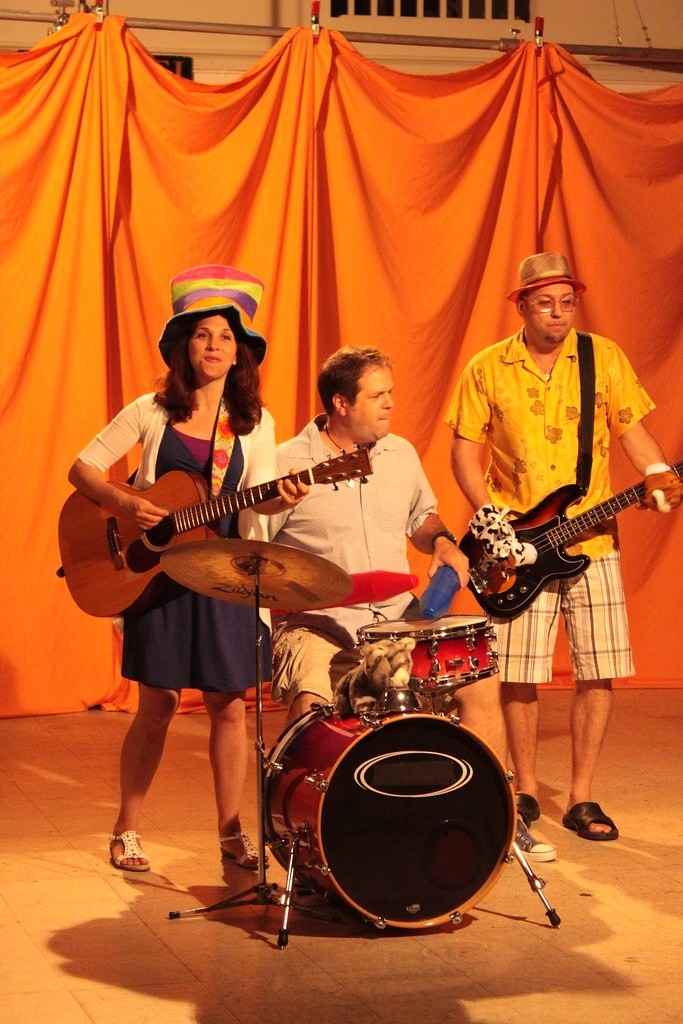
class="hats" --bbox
[506,251,587,303]
[158,264,267,370]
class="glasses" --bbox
[525,297,578,312]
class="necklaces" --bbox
[526,347,561,380]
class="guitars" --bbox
[458,460,680,617]
[57,443,374,617]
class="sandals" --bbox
[108,830,151,872]
[217,832,269,870]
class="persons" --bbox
[67,262,310,871]
[441,251,683,842]
[274,344,556,887]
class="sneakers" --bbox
[514,818,557,861]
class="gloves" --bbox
[467,503,538,571]
[634,463,683,514]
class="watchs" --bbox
[431,531,457,544]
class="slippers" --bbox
[514,793,540,829]
[561,801,619,841]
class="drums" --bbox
[356,615,500,692]
[262,702,517,930]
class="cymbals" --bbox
[160,538,355,612]
